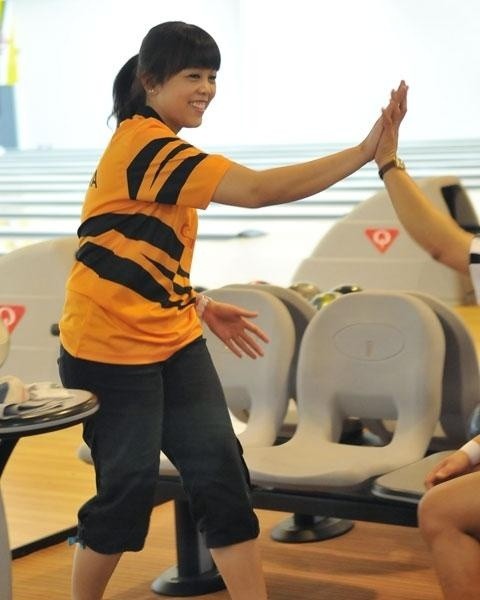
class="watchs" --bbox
[380,156,405,181]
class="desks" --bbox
[0,383,101,600]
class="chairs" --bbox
[80,278,480,595]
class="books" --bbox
[0,374,69,418]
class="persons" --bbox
[55,21,408,600]
[377,88,479,599]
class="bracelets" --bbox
[195,293,212,319]
[460,439,478,467]
